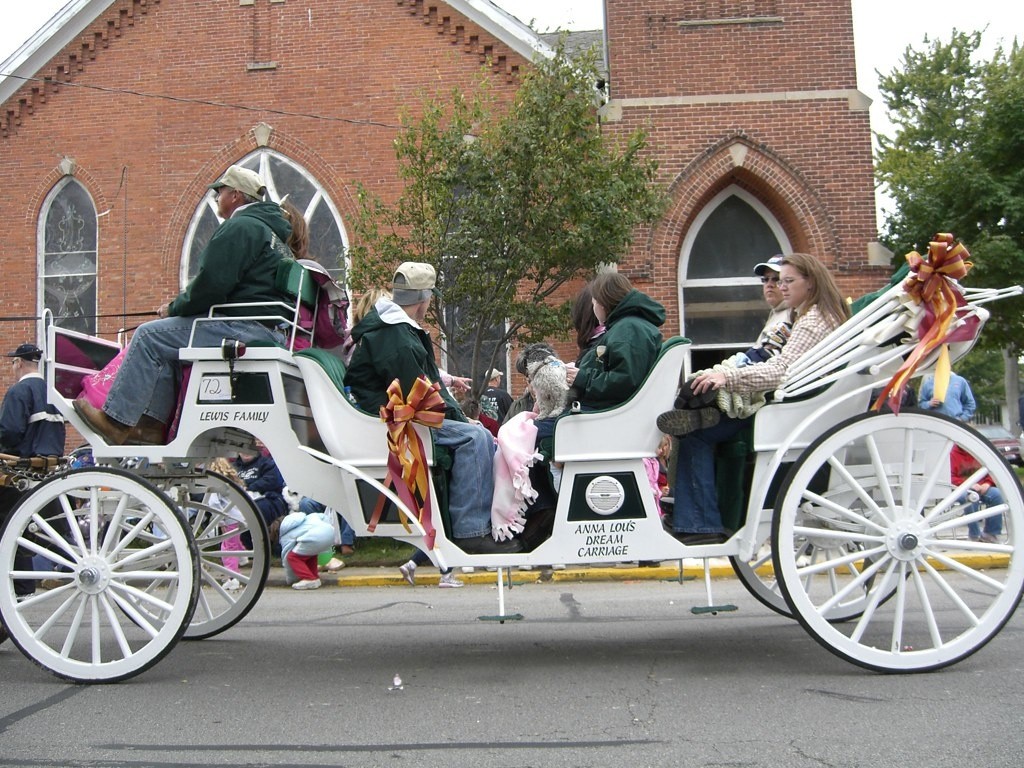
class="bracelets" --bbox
[450,375,455,387]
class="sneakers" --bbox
[291,579,323,590]
[222,579,240,590]
[439,573,462,587]
[72,398,129,446]
[239,557,248,566]
[399,562,415,586]
[128,416,167,446]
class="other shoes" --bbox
[671,531,727,545]
[656,406,722,435]
[455,534,522,554]
[969,532,1004,544]
[673,379,718,409]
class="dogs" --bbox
[514,339,574,420]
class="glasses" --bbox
[777,276,807,285]
[12,359,18,364]
[761,276,779,283]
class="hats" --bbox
[484,368,503,380]
[392,261,443,297]
[207,164,266,201]
[753,254,785,275]
[8,344,44,363]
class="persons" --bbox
[396,271,673,588]
[916,362,978,423]
[655,252,865,545]
[69,165,310,448]
[948,438,1005,545]
[32,452,355,591]
[0,342,70,609]
[342,261,521,555]
[867,383,916,412]
[340,288,474,393]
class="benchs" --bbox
[715,253,929,452]
[540,336,691,461]
[292,349,452,465]
[179,258,321,365]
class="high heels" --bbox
[638,560,660,567]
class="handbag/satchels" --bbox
[323,506,342,545]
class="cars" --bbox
[974,423,1024,469]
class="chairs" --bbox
[934,501,1010,544]
